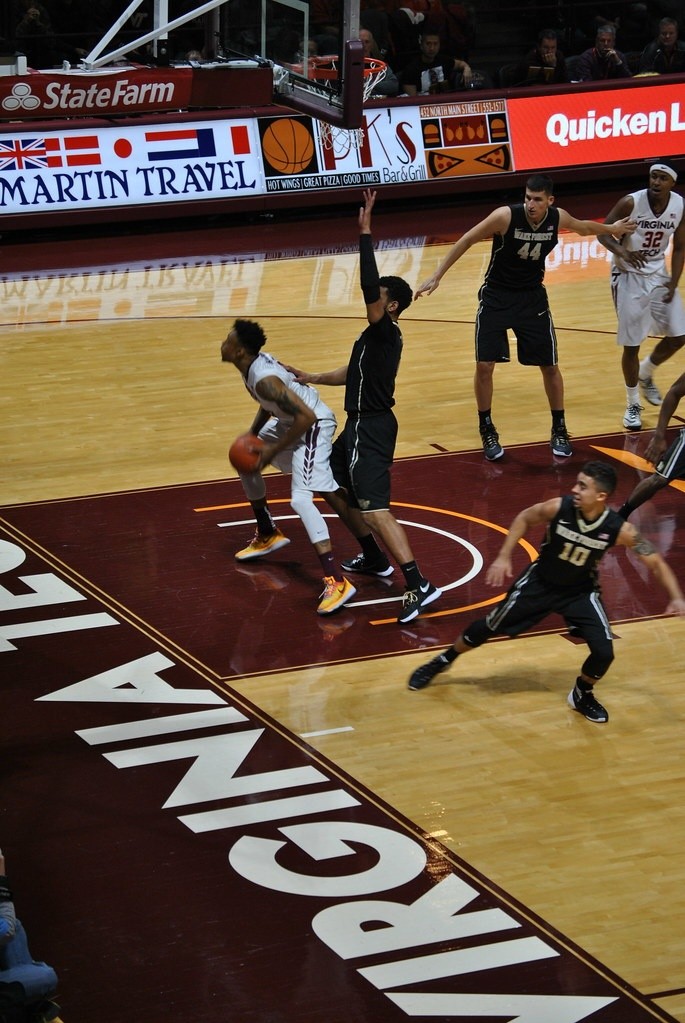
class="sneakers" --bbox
[398,578,441,624]
[478,426,504,459]
[317,575,356,613]
[399,629,439,649]
[319,618,356,640]
[234,526,290,560]
[408,655,446,691]
[551,427,572,456]
[235,563,289,592]
[568,676,610,722]
[639,376,662,407]
[339,556,393,577]
[340,571,394,592]
[552,453,570,475]
[483,456,504,483]
[622,400,644,429]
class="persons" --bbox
[597,159,685,429]
[407,461,685,723]
[0,0,685,96]
[610,372,685,522]
[220,318,358,613]
[414,174,639,460]
[284,188,442,623]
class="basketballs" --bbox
[229,436,265,474]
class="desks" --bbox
[0,58,274,123]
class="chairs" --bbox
[454,51,643,91]
